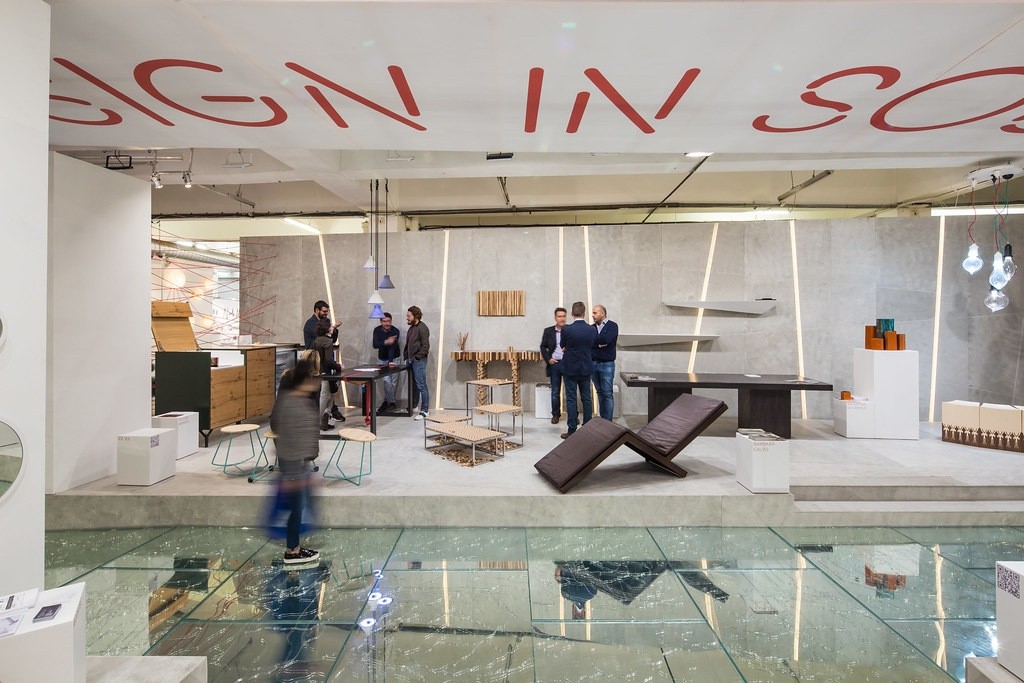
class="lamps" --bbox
[149,170,164,190]
[362,178,396,320]
[356,569,394,683]
[181,169,194,188]
[962,160,1023,313]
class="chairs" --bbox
[535,393,729,494]
[553,561,730,605]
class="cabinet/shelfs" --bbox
[854,349,919,440]
[151,300,301,447]
[616,298,777,349]
[865,544,920,577]
[148,555,223,631]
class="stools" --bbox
[465,378,515,437]
[423,413,472,443]
[211,423,377,488]
[470,403,524,453]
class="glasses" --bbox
[318,307,330,314]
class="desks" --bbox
[450,350,545,417]
[619,371,834,438]
[320,363,413,440]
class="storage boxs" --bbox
[597,384,620,418]
[117,428,175,486]
[735,432,789,495]
[151,411,199,459]
[942,400,1024,453]
[535,383,553,418]
[832,397,874,439]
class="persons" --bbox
[303,300,346,423]
[372,312,402,413]
[402,306,430,421]
[560,301,598,440]
[555,566,563,583]
[311,318,336,430]
[591,304,619,424]
[539,306,571,426]
[259,350,325,565]
[560,569,598,619]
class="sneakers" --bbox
[283,548,319,564]
[329,405,345,421]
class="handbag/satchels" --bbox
[326,365,341,394]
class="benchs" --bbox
[424,422,507,469]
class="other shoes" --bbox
[401,406,419,413]
[414,409,429,421]
[377,402,397,413]
[321,412,334,431]
[561,433,569,439]
[551,414,558,424]
